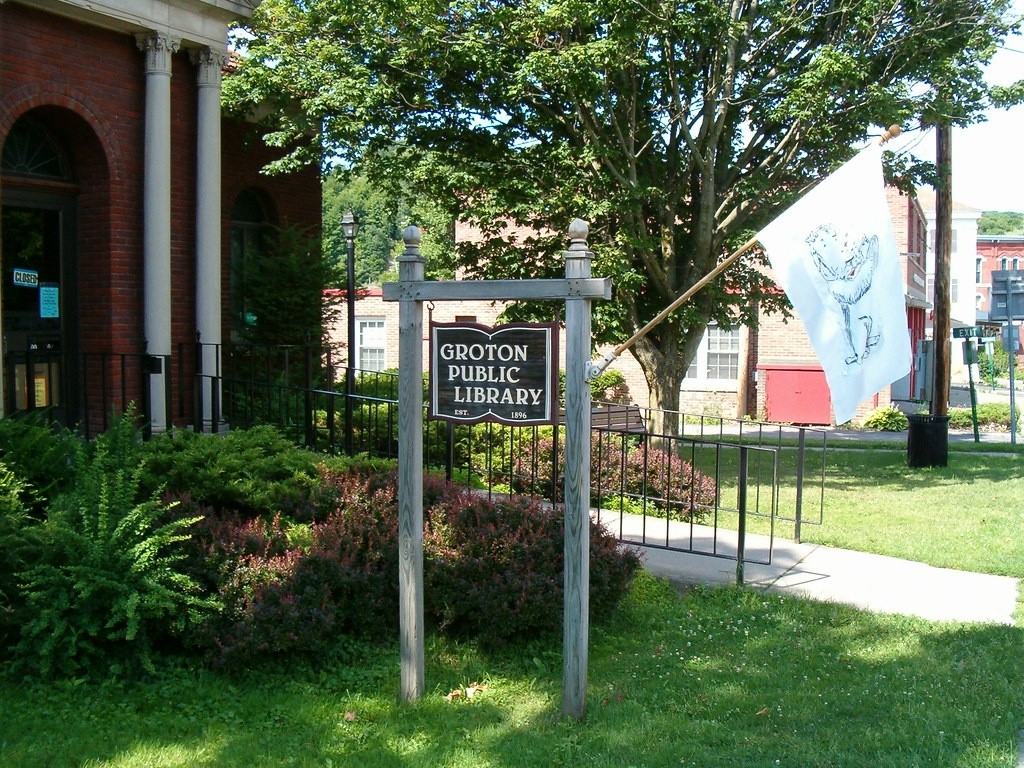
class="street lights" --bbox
[339,209,361,401]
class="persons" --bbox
[754,139,914,426]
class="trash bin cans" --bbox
[906,414,953,469]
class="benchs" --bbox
[557,403,648,446]
